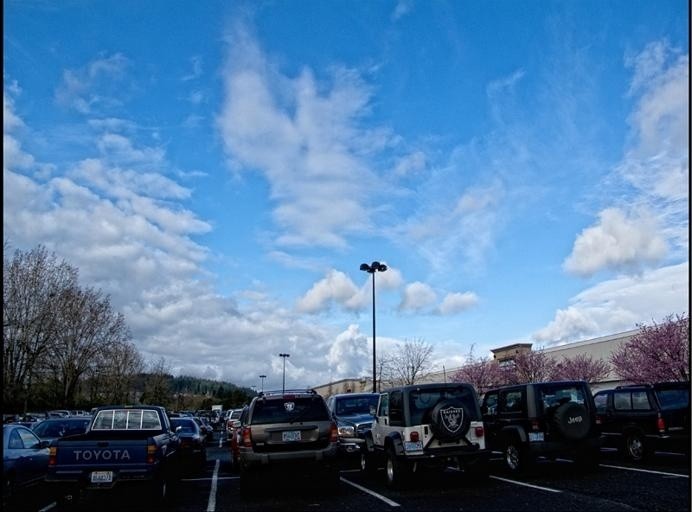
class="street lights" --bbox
[358,260,387,391]
[278,352,290,394]
[259,375,267,392]
[251,386,256,391]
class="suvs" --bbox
[474,378,603,471]
[356,381,489,489]
[327,391,381,458]
[590,380,690,464]
